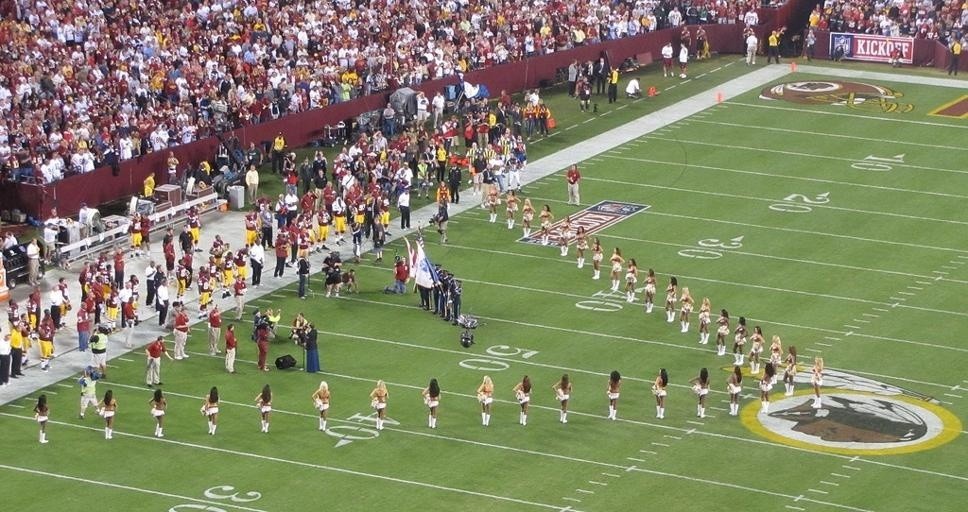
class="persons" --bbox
[802,0,968,76]
[811,356,823,408]
[1,1,800,440]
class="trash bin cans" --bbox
[229,185,244,209]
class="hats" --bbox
[432,263,462,294]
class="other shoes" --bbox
[419,304,459,327]
[41,227,383,387]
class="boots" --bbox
[523,227,531,238]
[654,400,771,419]
[732,352,743,365]
[645,301,653,313]
[507,219,515,229]
[39,405,618,444]
[680,320,689,332]
[542,235,548,246]
[576,256,584,268]
[560,245,568,256]
[610,280,619,292]
[784,383,794,396]
[698,332,708,344]
[666,310,675,322]
[626,291,634,303]
[591,269,600,279]
[716,344,725,355]
[490,213,497,223]
[771,375,777,385]
[749,361,761,374]
